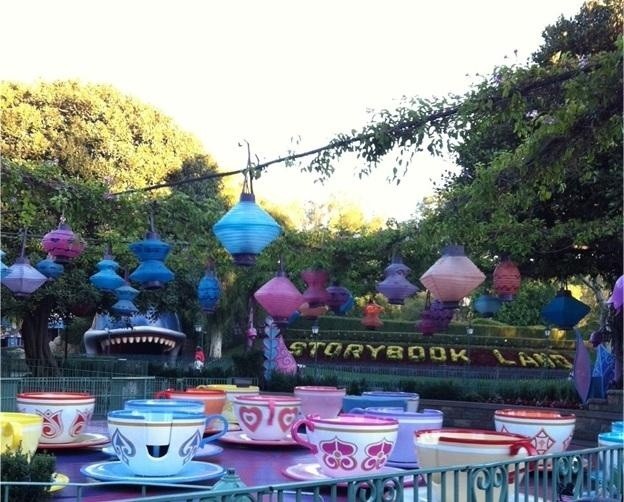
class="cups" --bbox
[104,406,230,478]
[595,420,624,476]
[1,410,43,471]
[413,426,539,502]
[495,408,579,461]
[125,383,444,459]
[18,389,93,440]
[291,411,402,479]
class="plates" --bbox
[385,486,543,502]
[81,460,223,484]
[46,472,73,494]
[383,462,420,469]
[530,456,588,474]
[102,441,226,459]
[279,462,426,490]
[39,432,108,449]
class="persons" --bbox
[193,346,204,373]
[193,356,204,373]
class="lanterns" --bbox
[537,288,591,332]
[377,258,419,305]
[254,270,354,327]
[199,272,221,314]
[44,224,83,266]
[113,283,139,313]
[362,303,385,331]
[475,294,503,317]
[420,247,486,336]
[91,255,125,293]
[0,251,64,298]
[493,261,521,300]
[213,192,282,268]
[130,232,173,289]
